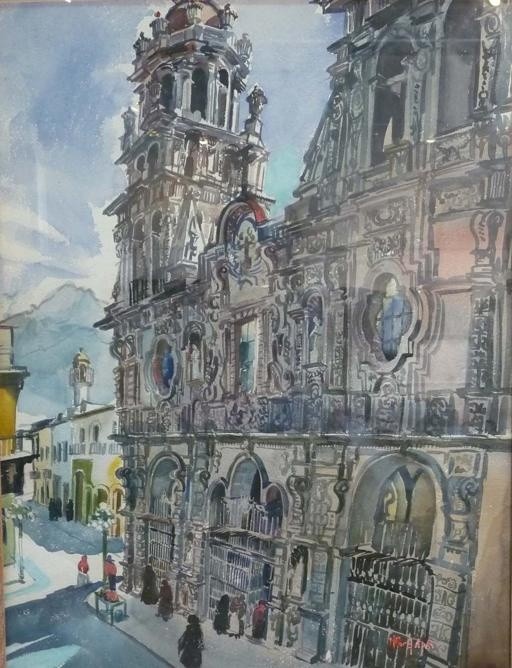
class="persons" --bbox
[154,580,175,619]
[212,593,301,646]
[105,555,118,591]
[46,497,73,522]
[138,566,159,605]
[77,553,94,585]
[177,614,204,668]
